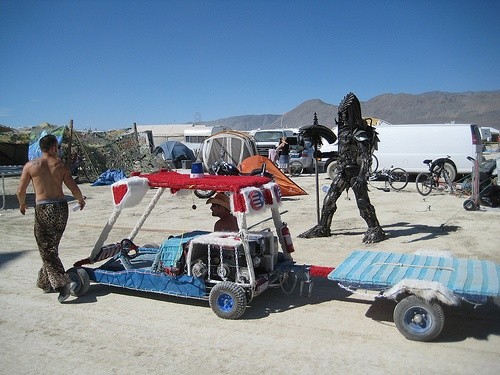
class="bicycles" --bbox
[273,155,455,195]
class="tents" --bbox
[27,120,309,199]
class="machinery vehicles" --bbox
[63,174,500,344]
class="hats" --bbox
[206,193,231,212]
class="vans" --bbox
[318,123,482,182]
[253,130,294,157]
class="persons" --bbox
[17,134,86,303]
[206,192,239,232]
[276,138,292,176]
[298,93,386,244]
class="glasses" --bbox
[212,204,221,207]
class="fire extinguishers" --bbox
[280,222,295,253]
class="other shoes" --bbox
[44,286,54,293]
[58,286,71,303]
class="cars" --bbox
[291,141,315,171]
